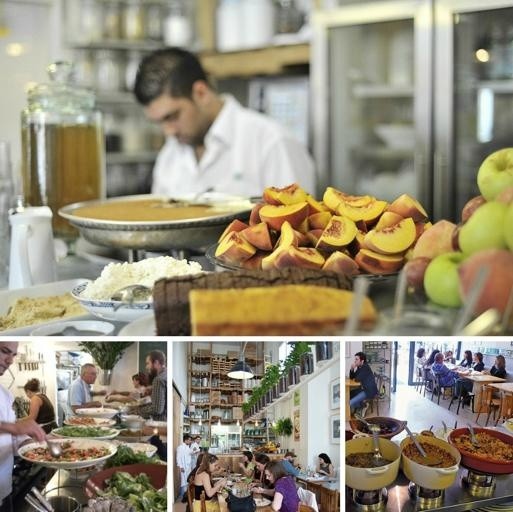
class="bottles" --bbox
[306,464,315,476]
[226,466,229,472]
[0,141,22,271]
[20,58,105,252]
[190,409,208,419]
[191,425,205,434]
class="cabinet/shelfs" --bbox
[55,364,78,393]
[242,428,274,447]
[195,0,311,80]
[184,341,266,450]
[61,39,167,165]
[15,359,47,392]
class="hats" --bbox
[286,452,297,457]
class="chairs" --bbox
[364,372,388,416]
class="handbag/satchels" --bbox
[229,494,253,512]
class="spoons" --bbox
[111,284,149,306]
[467,422,481,447]
[353,412,380,433]
[371,431,384,466]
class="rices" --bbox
[79,255,203,302]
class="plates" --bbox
[211,472,246,486]
[254,498,269,507]
[68,280,153,320]
[0,275,93,335]
[448,427,512,475]
[50,404,157,440]
[120,440,157,457]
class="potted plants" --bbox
[241,341,332,422]
[77,341,134,386]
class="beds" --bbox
[184,468,341,512]
[416,360,513,427]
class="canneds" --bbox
[202,377,209,387]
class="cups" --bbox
[468,368,473,375]
[246,429,264,435]
[37,495,80,511]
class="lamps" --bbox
[225,341,258,380]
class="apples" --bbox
[407,147,513,316]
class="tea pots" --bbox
[8,202,58,285]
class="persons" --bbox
[315,452,334,478]
[23,378,58,436]
[415,347,506,405]
[126,348,166,422]
[106,372,151,409]
[131,43,317,206]
[67,364,104,419]
[347,351,379,418]
[236,450,302,512]
[175,433,228,512]
[0,340,46,511]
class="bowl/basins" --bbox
[344,432,400,491]
[16,438,115,470]
[29,320,115,335]
[402,432,461,488]
[349,416,408,438]
[57,192,259,249]
[84,463,168,498]
[204,240,427,284]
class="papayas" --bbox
[214,182,432,276]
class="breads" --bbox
[188,286,377,335]
[152,268,353,335]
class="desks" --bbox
[345,376,365,421]
